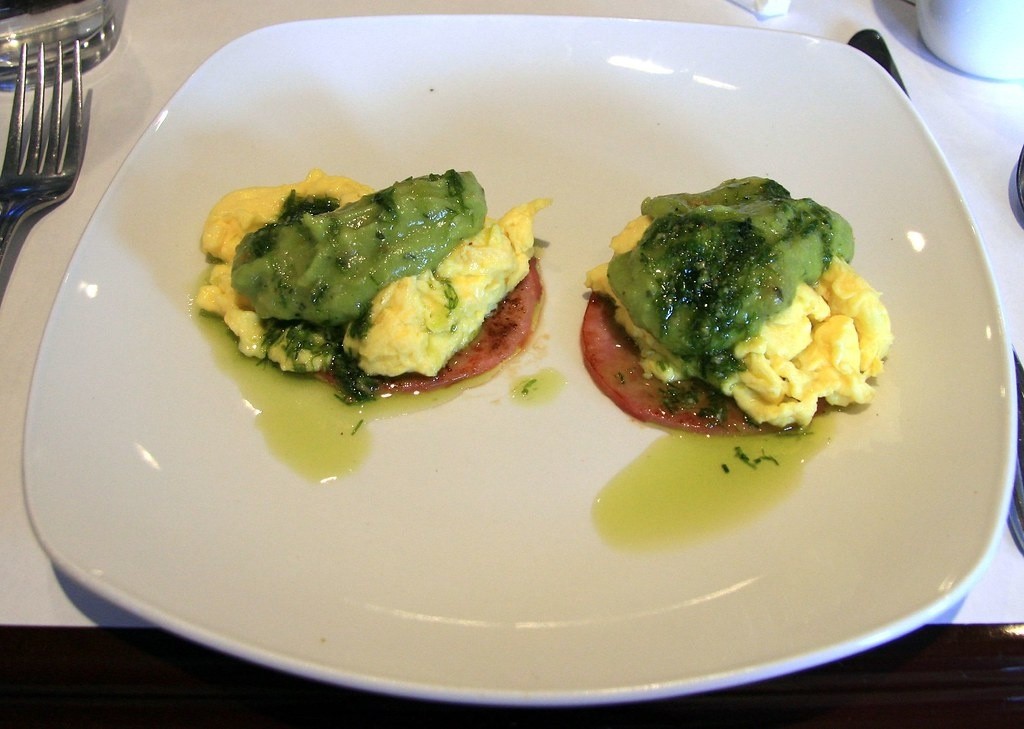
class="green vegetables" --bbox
[239,167,466,432]
[636,180,834,472]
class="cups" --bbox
[915,0,1022,80]
[1,1,122,89]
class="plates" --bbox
[19,14,1015,710]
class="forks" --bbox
[1,36,88,266]
